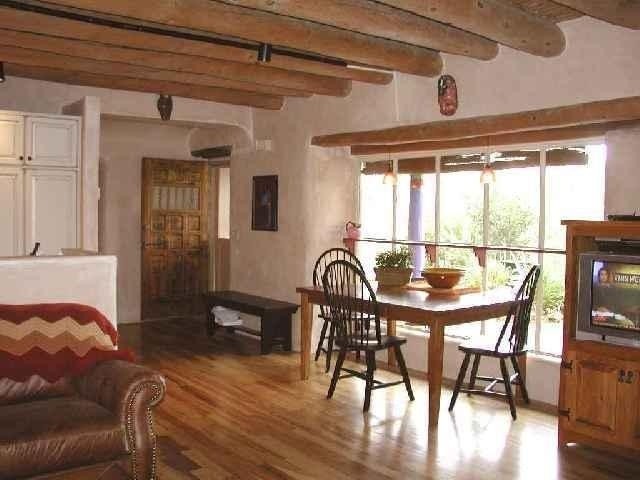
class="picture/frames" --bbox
[251,175,280,232]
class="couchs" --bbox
[0,303,166,480]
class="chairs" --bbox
[323,260,416,413]
[448,264,541,420]
[313,248,378,373]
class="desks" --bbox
[294,280,528,427]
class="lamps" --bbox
[383,149,398,186]
[481,136,496,184]
[410,175,422,189]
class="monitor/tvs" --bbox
[576,252,640,347]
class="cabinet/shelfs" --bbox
[1,109,83,254]
[557,216,640,462]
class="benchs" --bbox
[200,291,300,355]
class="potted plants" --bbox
[373,246,415,287]
